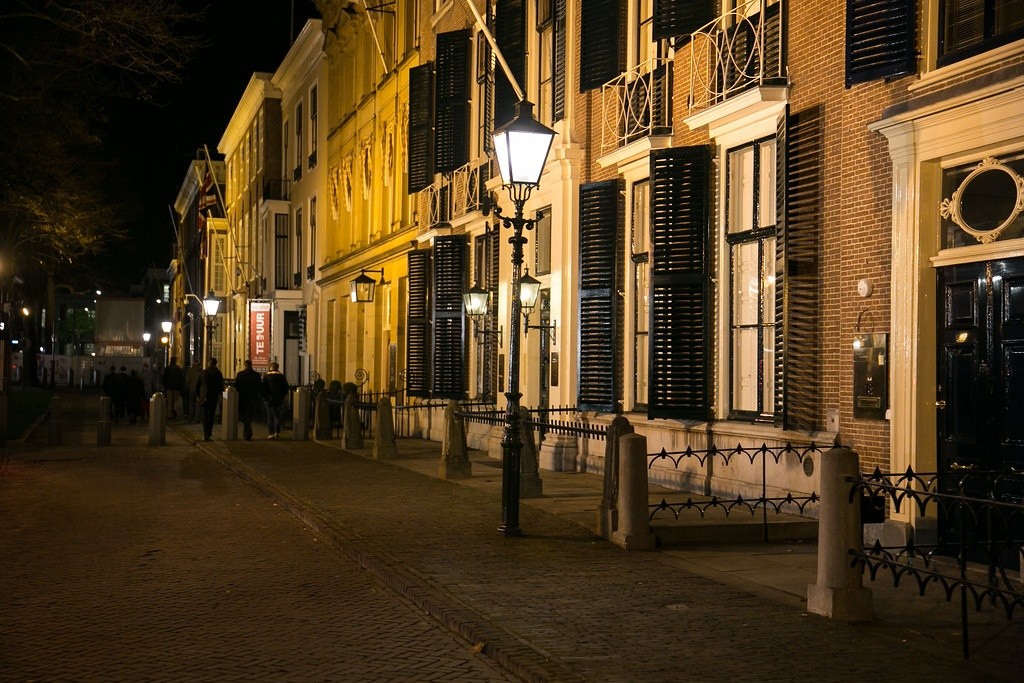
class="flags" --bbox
[197,155,218,261]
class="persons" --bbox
[195,358,225,441]
[234,359,262,441]
[261,363,290,439]
[102,357,203,425]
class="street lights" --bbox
[160,319,174,371]
[461,89,560,537]
[203,290,221,364]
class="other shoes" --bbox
[268,434,274,439]
[274,436,280,441]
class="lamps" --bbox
[461,281,503,349]
[349,267,386,303]
[521,263,556,347]
[235,268,241,276]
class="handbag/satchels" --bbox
[197,374,207,406]
